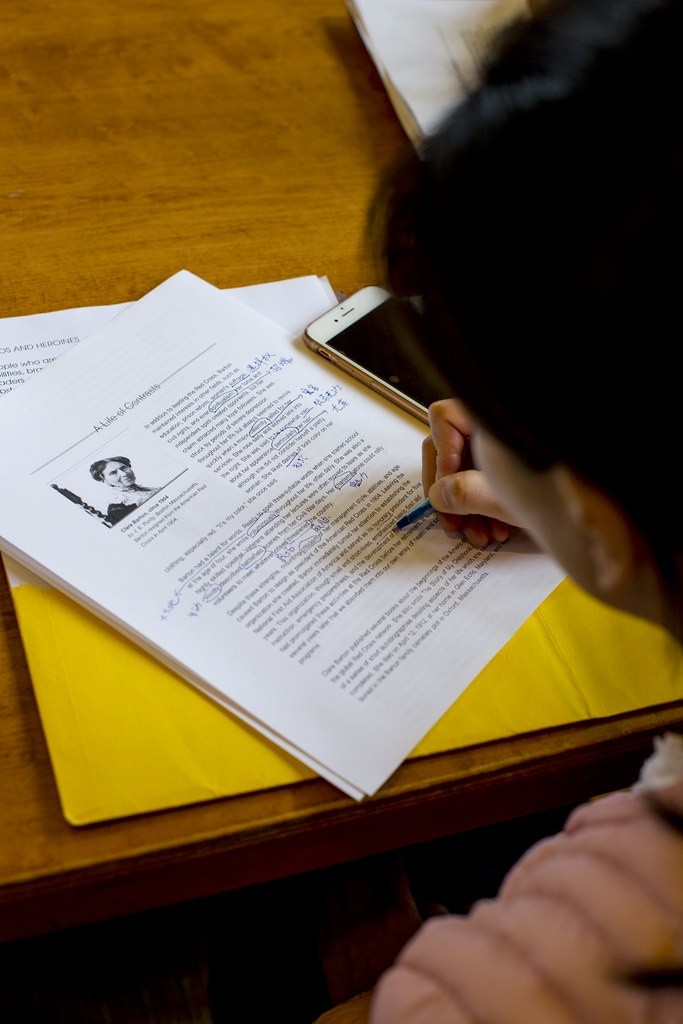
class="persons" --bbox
[315,0,683,1024]
[90,456,160,526]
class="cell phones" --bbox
[300,284,447,427]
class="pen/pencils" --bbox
[392,498,434,531]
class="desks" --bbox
[0,0,683,946]
